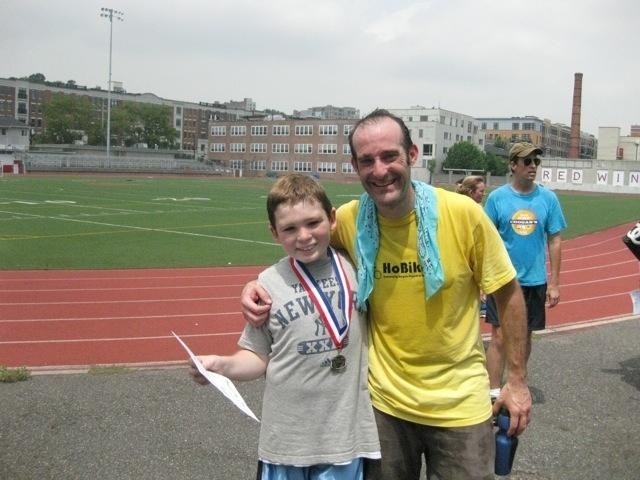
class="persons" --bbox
[456,170,486,205]
[238,107,534,480]
[186,170,384,480]
[477,139,569,428]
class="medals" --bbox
[331,355,348,370]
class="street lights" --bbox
[98,5,126,170]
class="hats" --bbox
[508,141,543,160]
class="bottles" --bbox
[493,411,519,476]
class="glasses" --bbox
[517,158,541,166]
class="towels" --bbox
[355,177,440,316]
